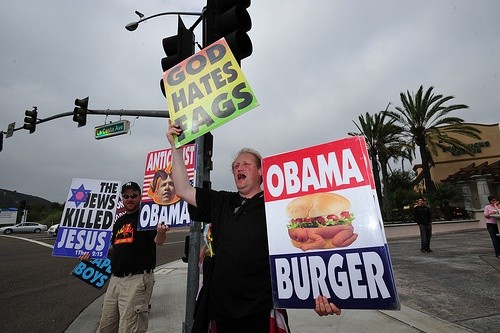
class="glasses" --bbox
[122,194,138,199]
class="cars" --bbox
[0,221,48,235]
[47,223,59,237]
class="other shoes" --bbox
[421,248,433,253]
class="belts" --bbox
[113,267,154,278]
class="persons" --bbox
[166,119,341,333]
[157,174,175,202]
[414,198,433,253]
[78,182,170,333]
[200,224,213,262]
[288,225,358,251]
[484,196,500,257]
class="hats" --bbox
[121,181,141,194]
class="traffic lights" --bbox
[206,0,253,69]
[73,96,89,128]
[160,14,196,99]
[23,109,37,134]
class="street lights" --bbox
[125,6,206,333]
